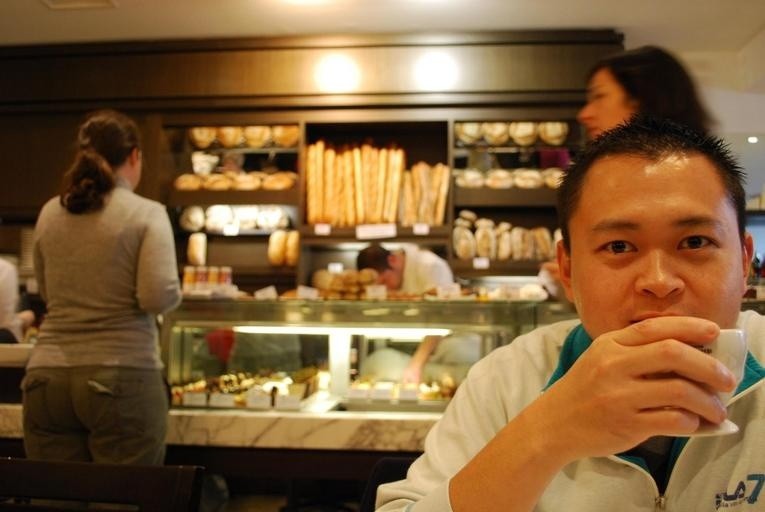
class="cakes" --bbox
[172,370,319,407]
[421,376,455,400]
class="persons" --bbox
[374,109,765,512]
[573,45,714,142]
[356,240,480,403]
[19,108,187,512]
[0,254,37,343]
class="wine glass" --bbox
[661,328,747,439]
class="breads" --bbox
[175,171,299,191]
[311,268,378,300]
[266,229,286,266]
[452,210,564,262]
[306,139,451,229]
[453,121,570,148]
[187,232,208,266]
[181,204,298,236]
[186,124,300,149]
[286,229,300,266]
[455,169,566,189]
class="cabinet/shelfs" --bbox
[160,114,589,292]
